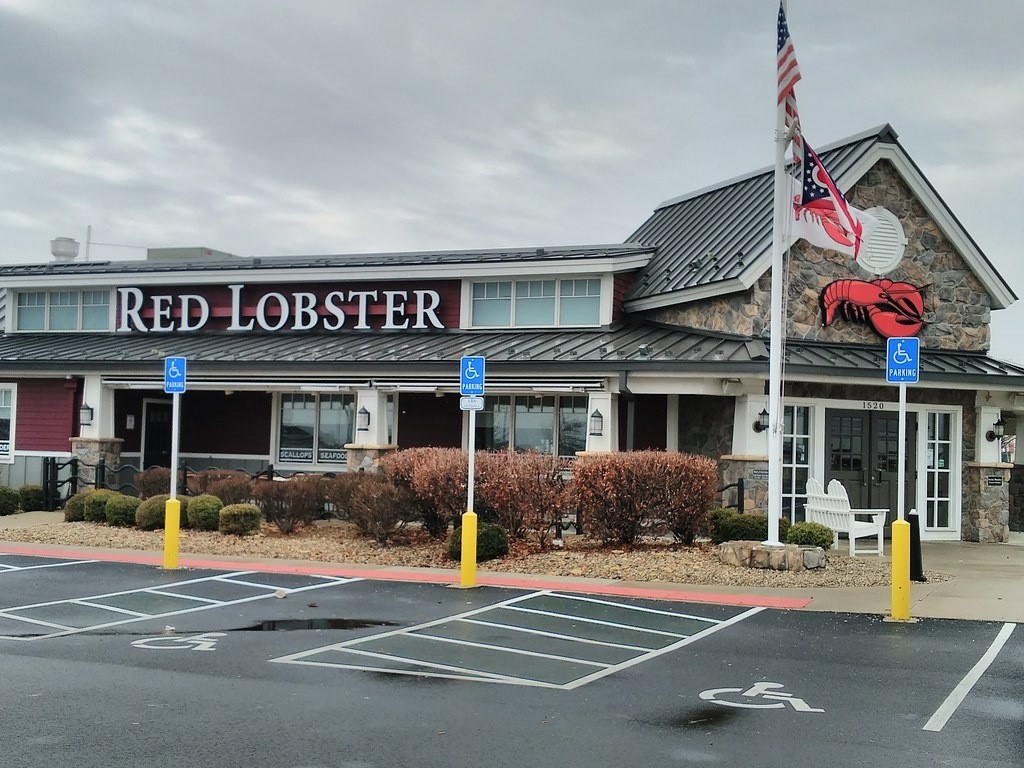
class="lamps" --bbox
[721,378,743,397]
[79,403,94,426]
[588,408,604,437]
[356,405,370,432]
[986,418,1004,443]
[753,404,770,433]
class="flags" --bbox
[777,1,802,163]
[800,135,863,242]
[781,174,876,259]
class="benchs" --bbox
[803,477,890,558]
